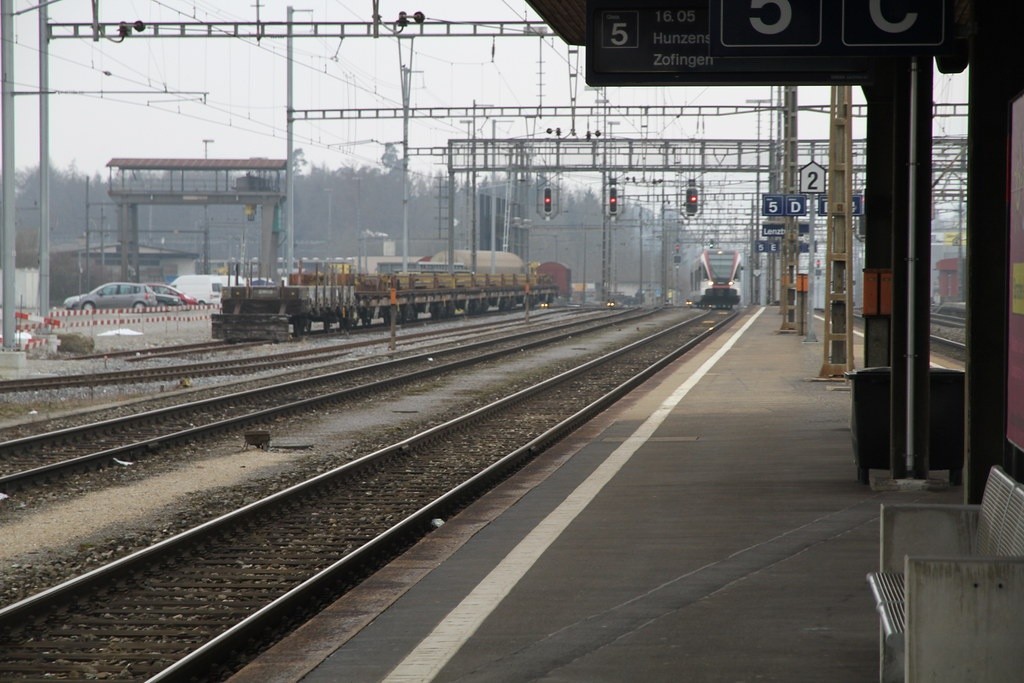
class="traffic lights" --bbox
[610,188,617,214]
[686,188,698,216]
[543,186,553,215]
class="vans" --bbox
[161,276,225,307]
[220,275,245,287]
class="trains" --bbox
[687,247,744,308]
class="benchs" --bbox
[864,464,1023,681]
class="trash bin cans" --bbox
[843,367,893,486]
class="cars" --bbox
[147,284,198,306]
[64,282,158,311]
[155,293,182,307]
